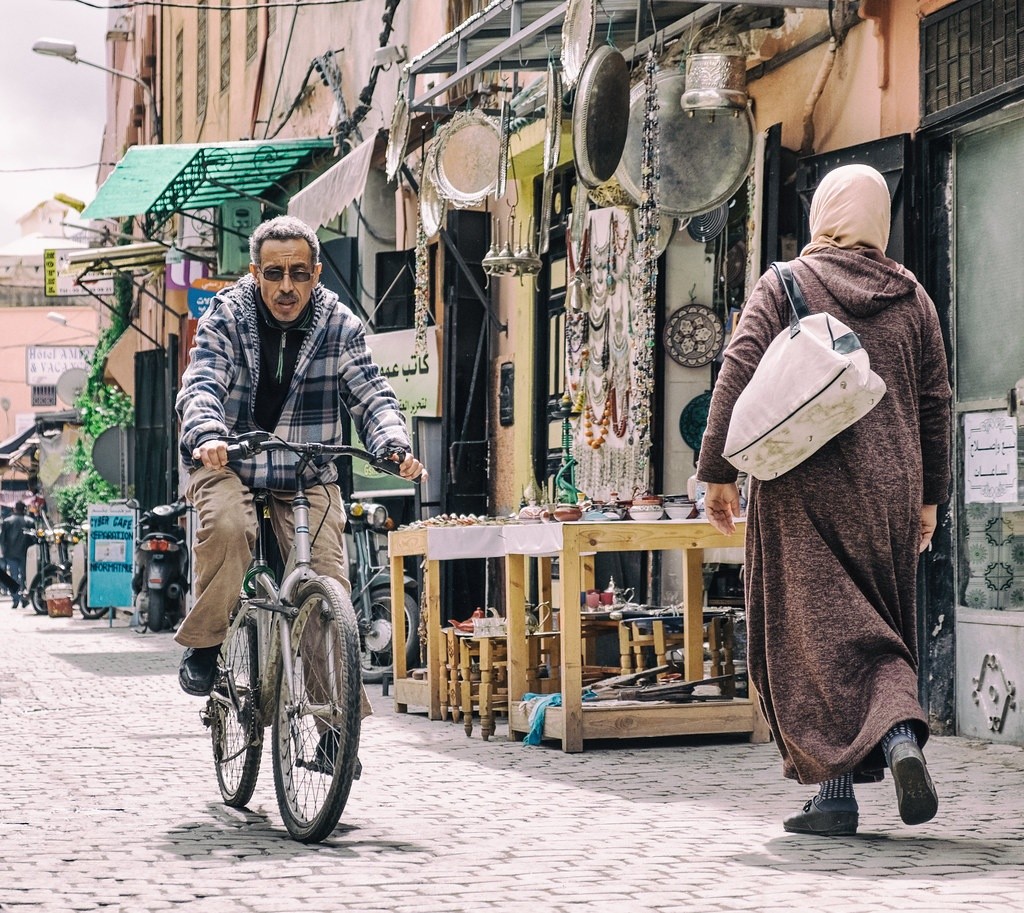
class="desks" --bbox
[503,516,773,754]
[388,525,596,721]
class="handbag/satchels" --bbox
[721,261,886,480]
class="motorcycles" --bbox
[26,524,113,620]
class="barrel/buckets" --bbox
[45,583,72,616]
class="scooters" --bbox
[127,498,197,634]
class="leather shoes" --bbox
[783,795,861,833]
[180,646,223,694]
[890,742,939,826]
[316,728,363,780]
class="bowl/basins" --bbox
[664,507,693,520]
[553,504,582,522]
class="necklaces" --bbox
[564,220,655,501]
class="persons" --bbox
[695,163,953,834]
[174,213,430,780]
[0,499,53,608]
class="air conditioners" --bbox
[214,199,262,275]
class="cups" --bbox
[587,594,599,608]
[472,618,504,636]
[600,593,613,605]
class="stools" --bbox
[439,611,648,741]
[619,609,736,697]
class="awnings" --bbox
[0,426,37,477]
[286,120,509,332]
[68,242,186,352]
[78,138,334,239]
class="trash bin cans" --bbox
[44,583,74,618]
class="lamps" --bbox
[106,16,131,41]
[55,193,121,232]
[166,215,181,264]
[47,312,100,339]
[32,42,161,136]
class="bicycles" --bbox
[199,429,421,843]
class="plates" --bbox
[385,90,410,183]
[680,394,711,451]
[689,201,728,242]
[559,0,598,84]
[664,305,725,368]
[719,241,747,292]
[427,108,502,210]
[419,154,446,238]
[728,184,749,226]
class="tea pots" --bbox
[448,607,484,632]
[604,576,634,609]
[489,600,551,635]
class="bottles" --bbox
[629,500,664,520]
[519,501,542,523]
[606,493,626,519]
[576,493,585,506]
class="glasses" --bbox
[259,265,315,281]
